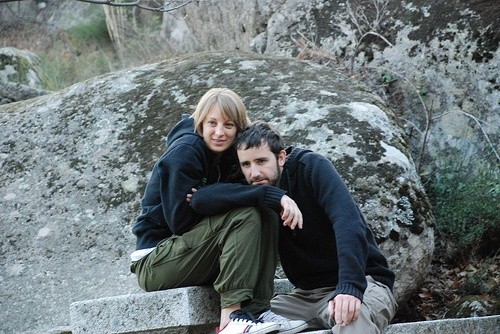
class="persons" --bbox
[130,87,310,334]
[190,122,398,334]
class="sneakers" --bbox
[218,308,280,334]
[256,310,308,334]
